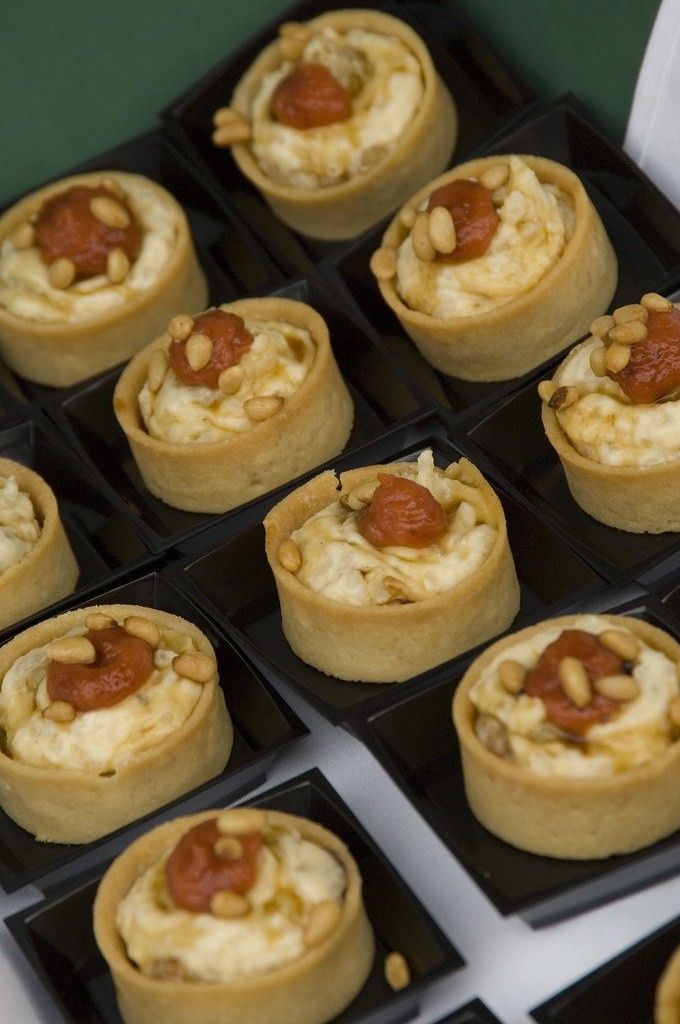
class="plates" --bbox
[17,766,469,1024]
[318,92,680,414]
[345,589,680,931]
[183,412,628,716]
[1,127,306,406]
[41,273,438,554]
[528,918,680,1024]
[0,406,162,635]
[0,569,311,896]
[158,0,549,265]
[462,290,680,588]
